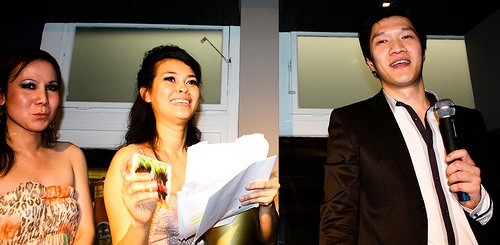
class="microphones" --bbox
[435,98,470,202]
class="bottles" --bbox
[92,180,112,245]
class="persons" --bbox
[319,6,500,245]
[0,48,96,245]
[103,44,280,245]
